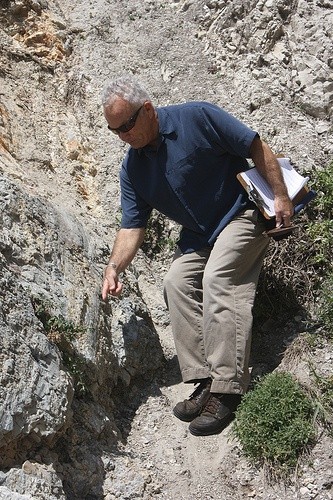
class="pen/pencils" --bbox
[276,190,317,229]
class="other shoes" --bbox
[172,382,217,423]
[192,393,243,437]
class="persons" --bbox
[101,81,293,435]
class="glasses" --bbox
[107,99,148,135]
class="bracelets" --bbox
[108,263,118,270]
